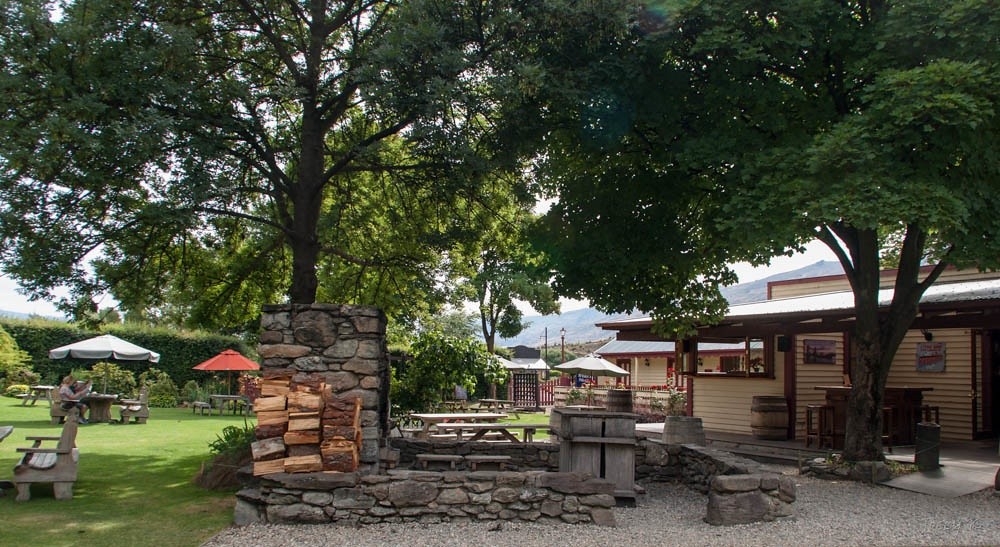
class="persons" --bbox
[59,373,91,425]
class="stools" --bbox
[805,404,833,449]
[417,453,463,468]
[466,455,512,471]
[910,406,939,442]
[881,407,893,452]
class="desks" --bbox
[85,394,118,422]
[410,414,508,433]
[436,423,551,443]
[476,399,520,420]
[565,405,606,411]
[814,385,933,446]
[23,386,55,407]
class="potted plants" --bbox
[749,358,764,372]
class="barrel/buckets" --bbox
[662,416,705,447]
[750,395,789,441]
[607,390,632,413]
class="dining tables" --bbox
[209,395,241,415]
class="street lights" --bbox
[539,327,549,381]
[560,328,566,363]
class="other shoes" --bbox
[78,417,88,424]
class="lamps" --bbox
[698,358,703,366]
[644,358,649,366]
[921,329,932,341]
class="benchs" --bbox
[463,406,524,410]
[193,402,211,416]
[402,429,519,439]
[240,403,254,416]
[13,385,150,502]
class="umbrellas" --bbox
[49,334,160,395]
[192,350,262,395]
[555,353,631,407]
